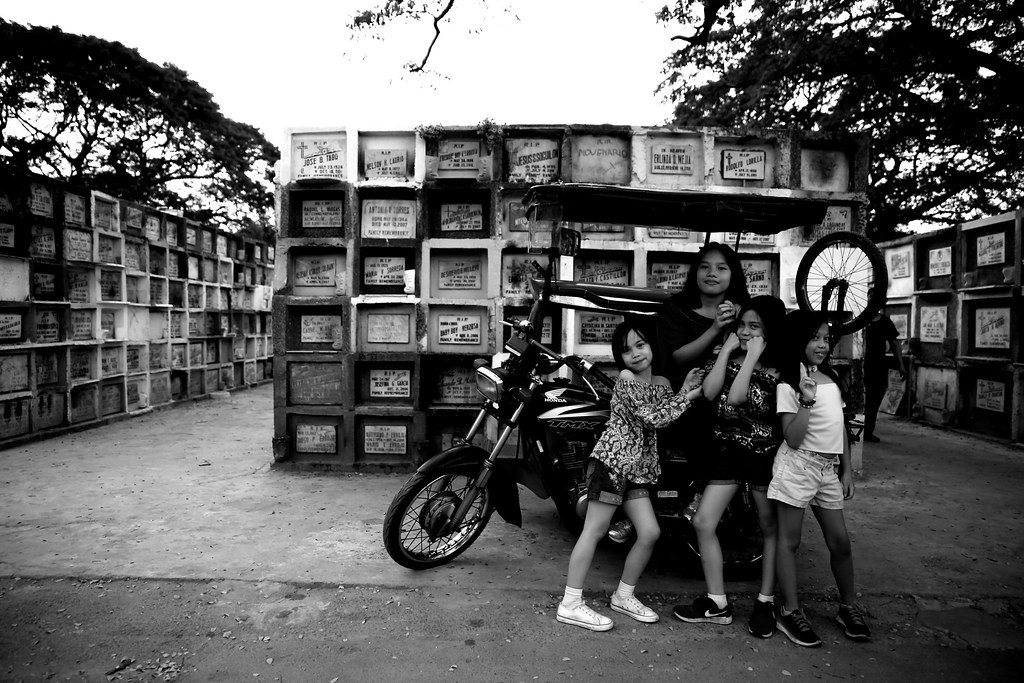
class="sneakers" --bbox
[775,604,823,647]
[609,591,660,623]
[835,604,872,641]
[555,598,614,632]
[748,599,777,638]
[672,592,732,625]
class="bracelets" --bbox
[799,398,816,407]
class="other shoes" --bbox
[681,492,703,525]
[862,433,881,443]
[607,519,634,544]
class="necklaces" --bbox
[808,365,819,371]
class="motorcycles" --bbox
[378,181,891,572]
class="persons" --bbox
[777,307,875,647]
[556,320,703,632]
[671,294,791,638]
[605,241,752,542]
[865,289,906,443]
